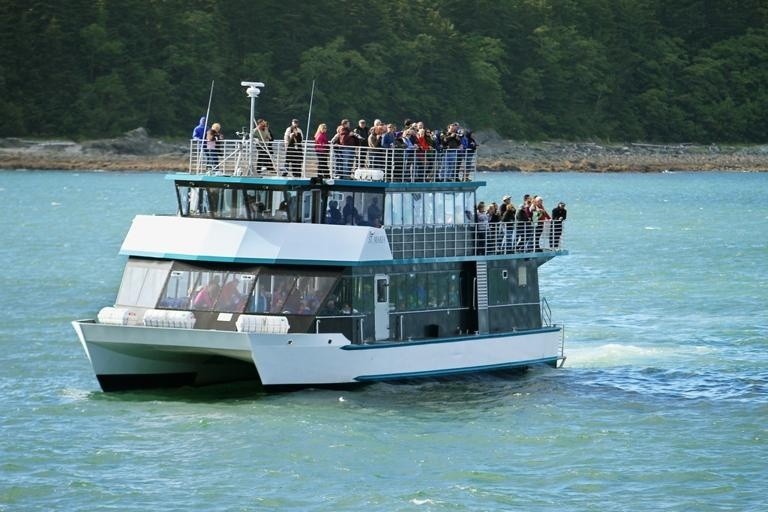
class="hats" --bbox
[503,195,511,201]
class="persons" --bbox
[388,271,459,310]
[241,191,297,221]
[323,195,383,227]
[464,192,567,255]
[193,112,480,181]
[190,275,352,316]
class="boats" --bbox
[71,81,568,394]
